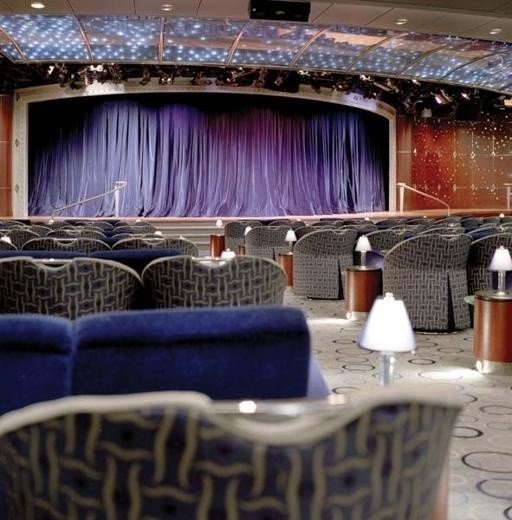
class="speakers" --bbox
[250,0,311,22]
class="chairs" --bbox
[225,217,511,333]
[0,213,290,309]
[0,307,332,401]
[0,380,476,518]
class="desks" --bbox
[474,288,511,375]
